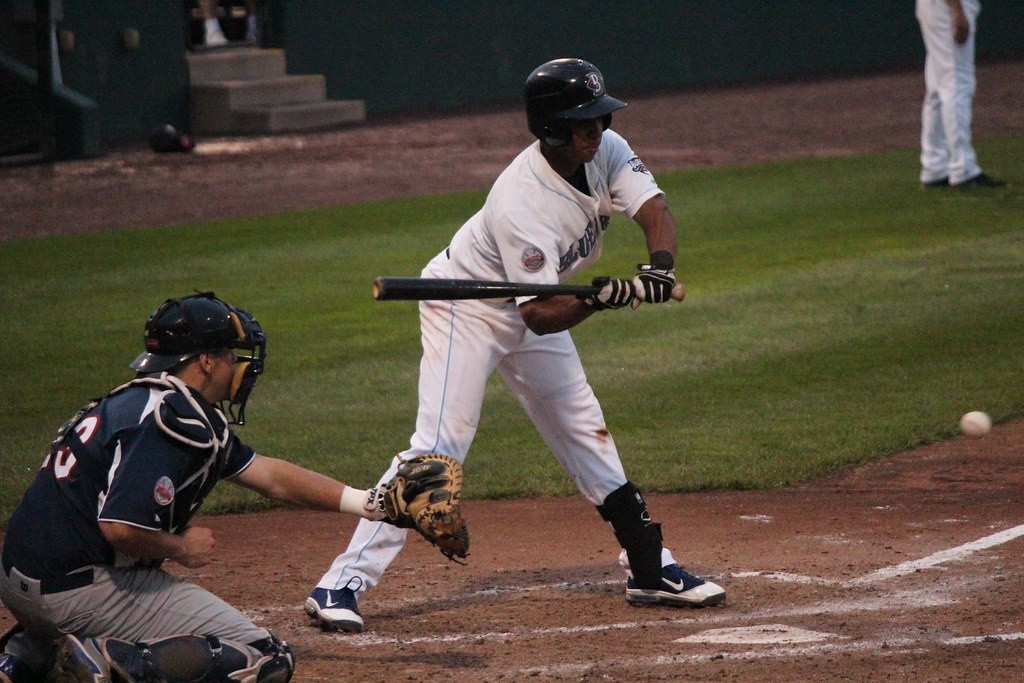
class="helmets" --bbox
[524,58,628,149]
[128,289,267,375]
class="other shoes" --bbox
[924,174,1003,193]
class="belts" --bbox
[3,548,93,594]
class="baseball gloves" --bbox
[379,453,471,568]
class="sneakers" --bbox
[67,632,154,683]
[626,565,728,611]
[302,575,364,631]
[0,633,57,681]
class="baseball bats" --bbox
[372,276,686,301]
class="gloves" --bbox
[577,273,634,311]
[633,251,677,303]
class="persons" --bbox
[199,0,264,46]
[915,0,1008,193]
[304,58,727,633]
[0,291,446,683]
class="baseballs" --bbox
[960,410,991,439]
